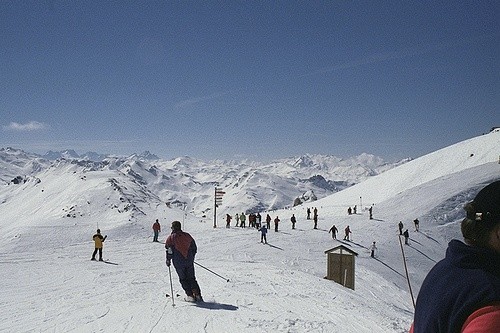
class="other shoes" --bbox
[194,294,204,304]
[261,240,267,244]
[153,239,159,242]
[91,257,96,261]
[99,257,103,261]
[184,295,196,303]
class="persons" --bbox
[369,206,374,219]
[352,204,357,214]
[329,225,339,239]
[165,221,203,303]
[410,180,500,333]
[402,229,410,245]
[265,213,271,229]
[258,225,267,244]
[225,214,232,226]
[234,213,239,227]
[313,207,318,229]
[291,214,296,229]
[90,229,104,262]
[348,207,352,215]
[343,225,352,241]
[274,216,280,232]
[151,219,161,242]
[306,208,311,220]
[371,241,377,258]
[239,212,246,228]
[248,212,262,229]
[414,219,420,232]
[398,221,403,235]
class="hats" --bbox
[464,179,500,221]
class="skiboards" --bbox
[165,292,205,304]
